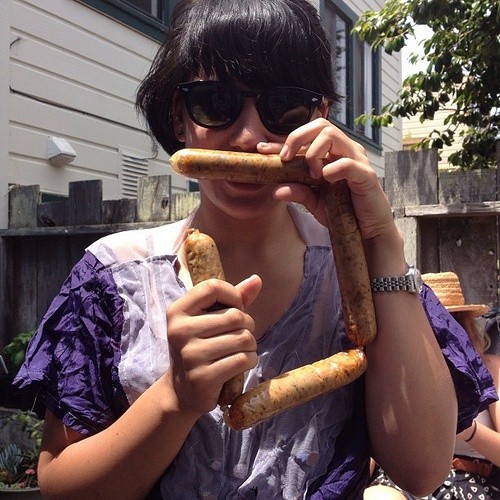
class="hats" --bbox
[420,270,489,315]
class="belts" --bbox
[450,457,490,478]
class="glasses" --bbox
[173,80,325,137]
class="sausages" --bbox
[168,148,378,433]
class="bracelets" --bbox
[465,420,476,442]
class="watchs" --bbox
[370,266,429,295]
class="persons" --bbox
[363,273,500,500]
[9,0,459,500]
